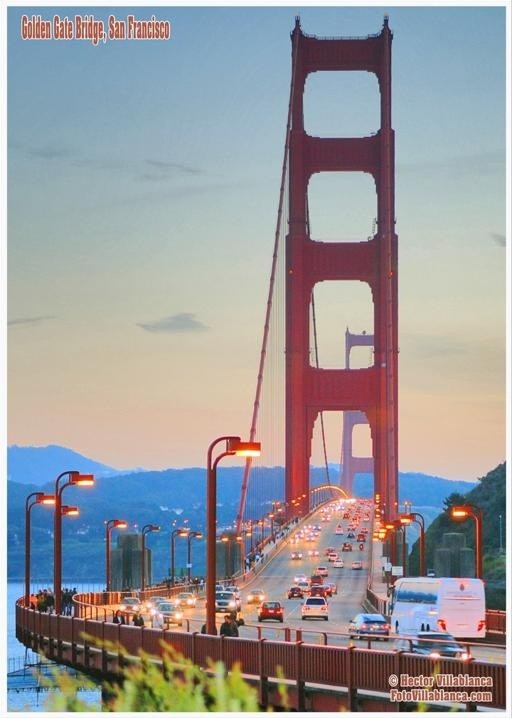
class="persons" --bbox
[220,615,239,637]
[133,610,144,626]
[244,550,264,572]
[113,609,125,625]
[201,623,217,636]
[152,607,164,629]
[126,576,206,601]
[29,586,78,616]
[230,608,238,621]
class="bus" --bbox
[387,577,487,646]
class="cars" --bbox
[391,631,472,659]
[300,596,329,621]
[287,498,373,599]
[215,585,265,614]
[348,614,390,641]
[256,600,285,624]
[118,592,197,626]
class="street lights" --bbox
[373,512,425,578]
[106,520,203,591]
[54,471,96,615]
[25,492,56,608]
[205,435,262,637]
[448,504,482,578]
[212,483,330,579]
